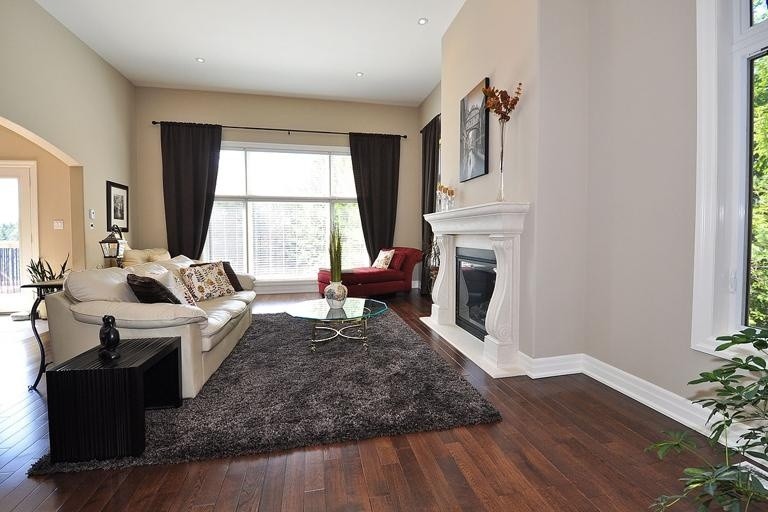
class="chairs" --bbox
[316,247,424,298]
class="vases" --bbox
[498,127,505,202]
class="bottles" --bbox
[440,187,448,212]
[446,189,455,210]
[435,184,443,212]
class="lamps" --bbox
[98,223,128,268]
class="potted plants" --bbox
[24,253,71,320]
[322,214,349,310]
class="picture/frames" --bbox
[459,77,489,183]
[106,180,129,232]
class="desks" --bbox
[21,278,66,394]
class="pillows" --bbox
[64,255,244,307]
[390,250,407,271]
[371,248,395,269]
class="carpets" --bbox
[26,308,503,478]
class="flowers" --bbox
[482,82,524,173]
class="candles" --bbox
[439,185,455,196]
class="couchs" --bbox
[44,260,257,399]
